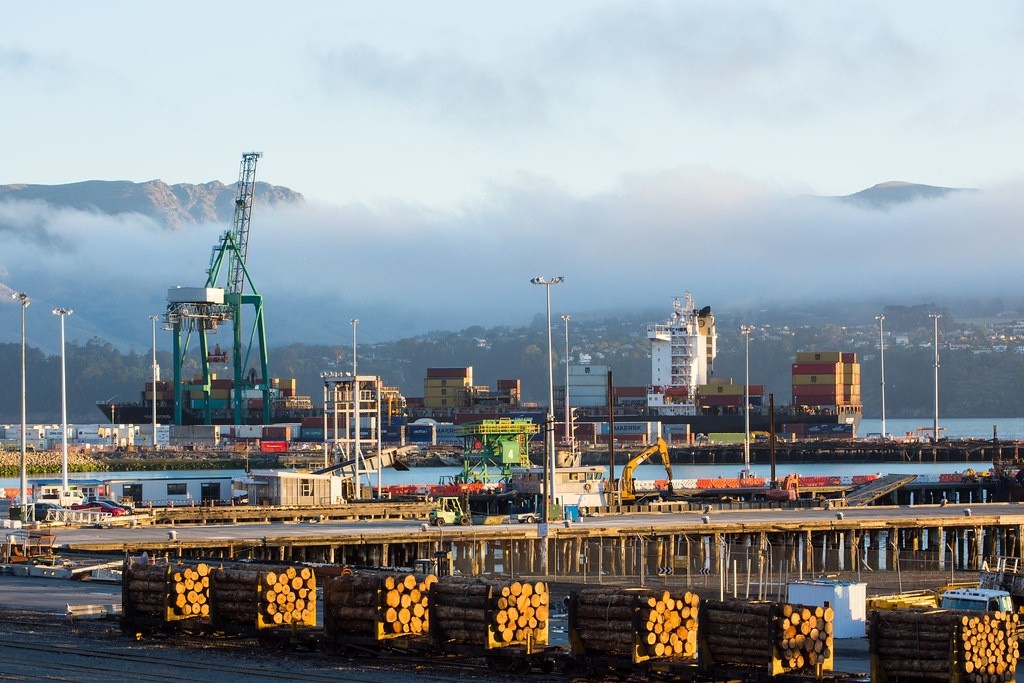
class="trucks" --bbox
[696,433,708,441]
[37,490,84,509]
[708,432,770,445]
[940,557,1024,612]
[510,512,543,523]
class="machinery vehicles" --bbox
[614,437,673,505]
[765,472,799,502]
[429,497,470,526]
[962,467,990,483]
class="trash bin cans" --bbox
[564,504,579,522]
[9,505,21,521]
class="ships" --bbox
[96,291,863,438]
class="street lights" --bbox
[530,276,564,504]
[149,314,159,446]
[12,292,31,523]
[738,325,755,478]
[561,314,571,445]
[929,314,943,442]
[350,318,360,499]
[51,307,73,491]
[875,313,886,437]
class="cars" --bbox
[71,501,126,517]
[35,502,62,518]
[82,496,117,505]
[89,499,132,515]
